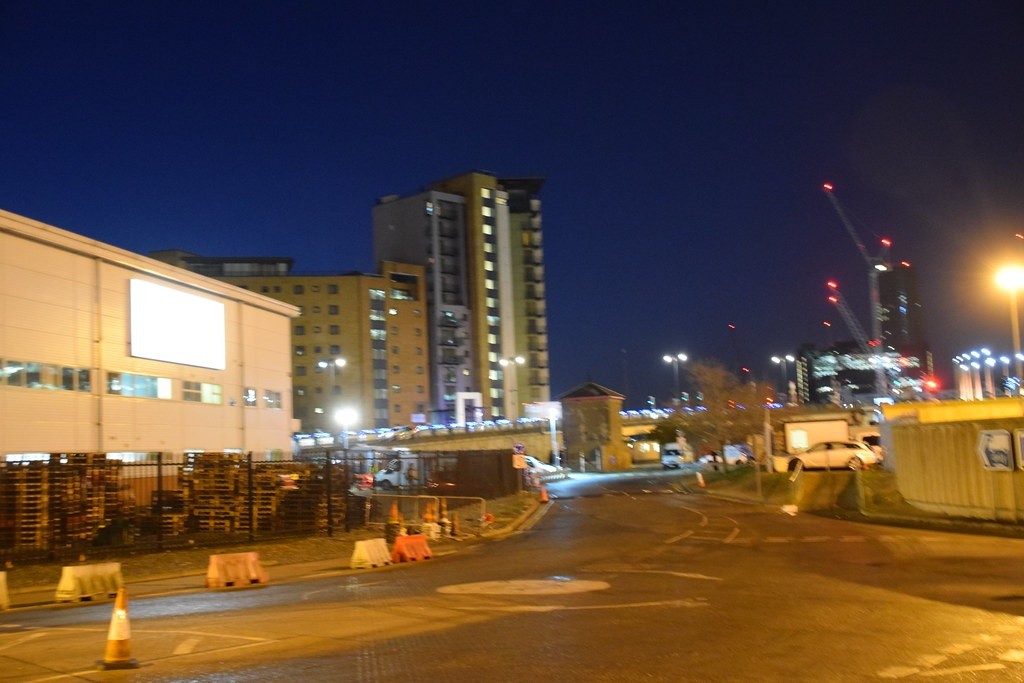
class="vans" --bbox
[374,454,420,490]
[661,444,684,469]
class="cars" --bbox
[788,440,876,470]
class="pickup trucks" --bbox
[705,443,748,465]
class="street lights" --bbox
[318,358,345,431]
[1001,267,1024,393]
[662,352,687,413]
[499,356,526,423]
[771,354,795,402]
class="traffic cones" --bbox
[449,512,460,536]
[538,486,549,502]
[387,500,401,525]
[422,504,435,521]
[96,587,139,670]
